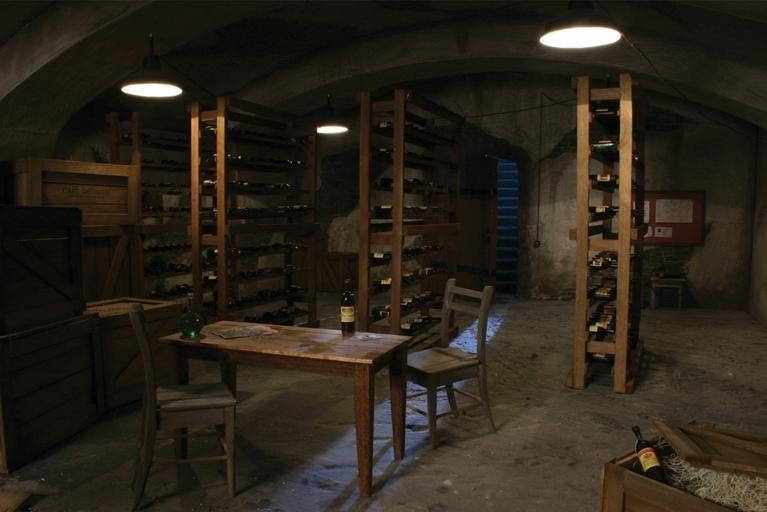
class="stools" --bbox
[649,270,684,310]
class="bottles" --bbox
[179,292,203,338]
[125,133,191,297]
[370,119,451,333]
[340,279,355,335]
[587,102,640,363]
[631,425,665,481]
[200,126,314,322]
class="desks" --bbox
[154,320,413,500]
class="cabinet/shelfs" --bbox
[357,82,462,353]
[0,148,189,475]
[566,71,648,394]
[189,93,320,334]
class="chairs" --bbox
[405,278,496,451]
[122,302,238,512]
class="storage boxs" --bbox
[598,421,767,511]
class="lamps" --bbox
[535,1,623,50]
[114,0,185,100]
